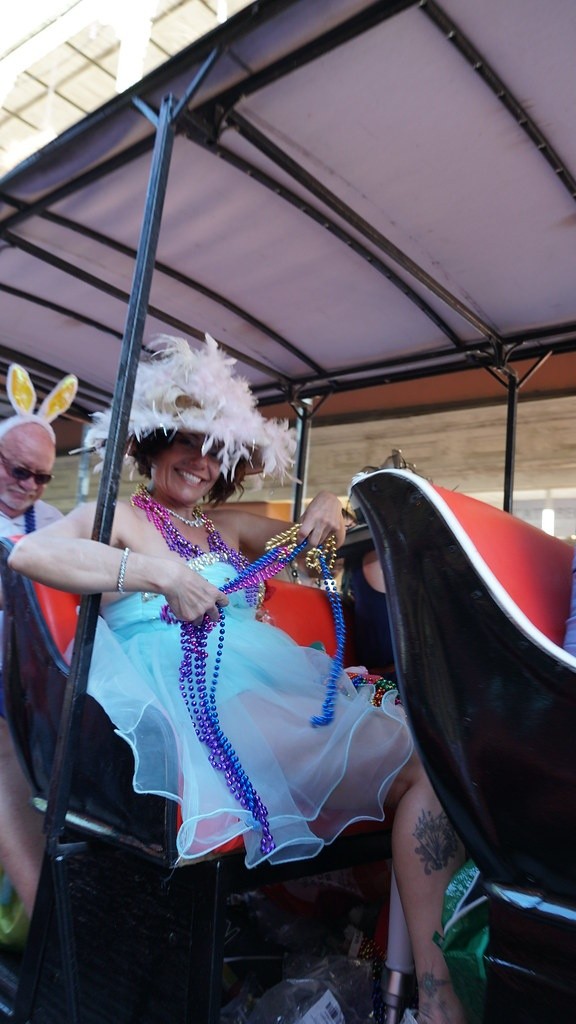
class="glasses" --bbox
[0,457,58,491]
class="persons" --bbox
[344,450,432,681]
[0,412,70,916]
[6,333,467,1024]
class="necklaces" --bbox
[159,523,343,853]
[23,504,36,533]
[133,483,260,606]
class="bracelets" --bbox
[117,547,130,594]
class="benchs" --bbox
[0,533,399,1024]
[348,467,576,1024]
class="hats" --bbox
[69,330,302,500]
